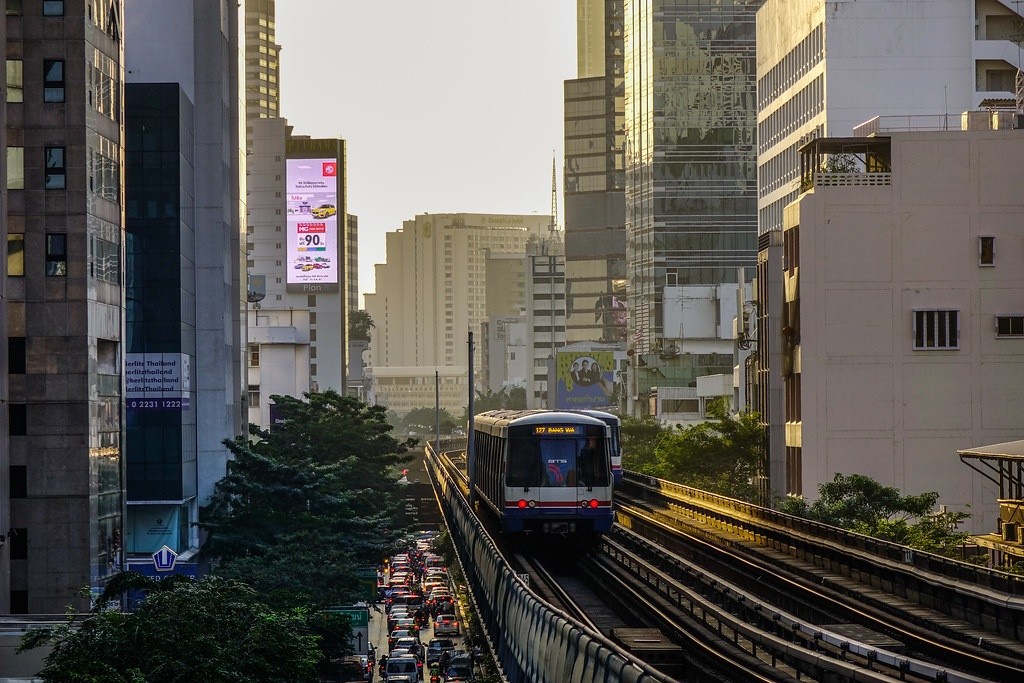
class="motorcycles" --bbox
[377,660,387,680]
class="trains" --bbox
[467,408,623,542]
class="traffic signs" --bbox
[313,606,369,655]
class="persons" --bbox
[570,360,580,384]
[590,363,600,384]
[578,360,592,386]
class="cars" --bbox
[352,642,378,679]
[378,531,476,683]
